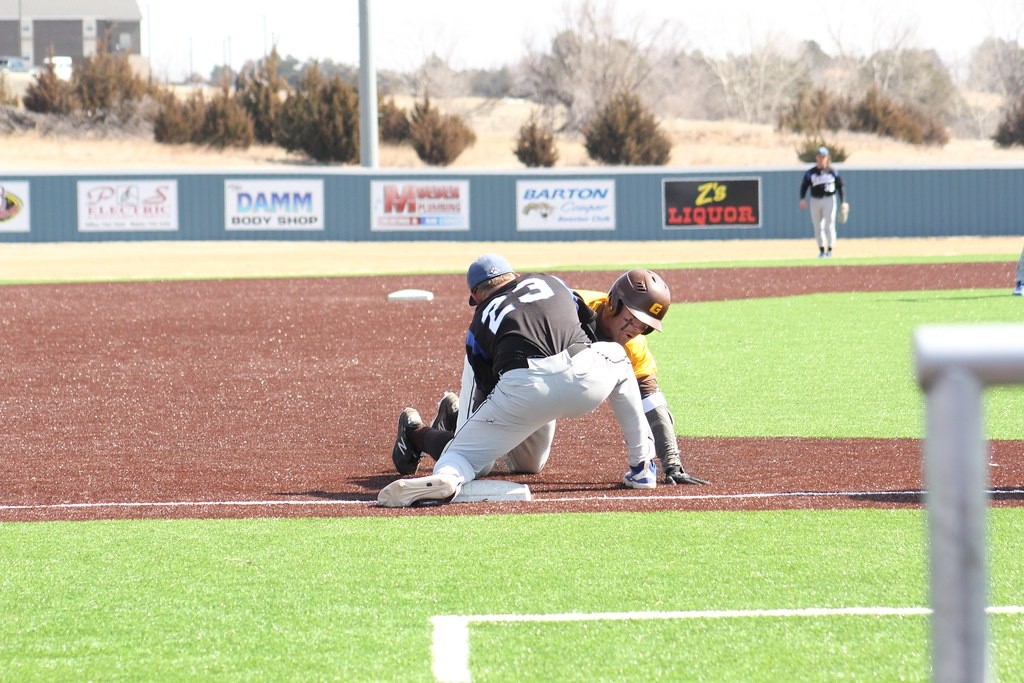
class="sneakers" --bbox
[378,472,458,508]
[430,392,459,432]
[392,408,424,476]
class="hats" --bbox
[815,146,830,155]
[467,253,515,306]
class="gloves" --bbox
[665,466,710,487]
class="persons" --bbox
[798,148,844,257]
[376,255,658,509]
[391,269,712,486]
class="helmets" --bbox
[607,269,671,335]
